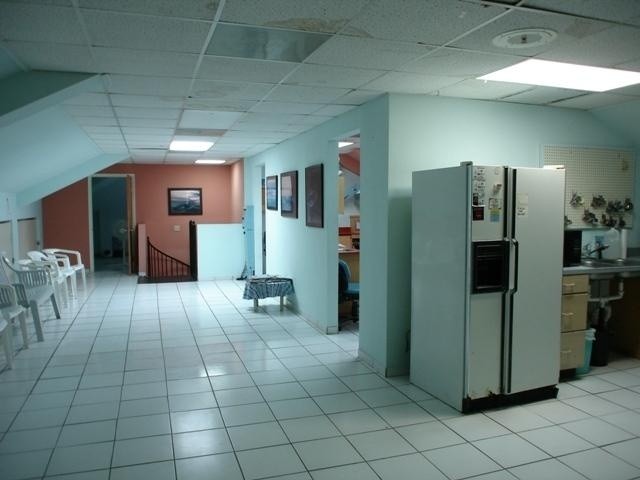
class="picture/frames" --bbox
[168,187,203,216]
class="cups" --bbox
[572,248,581,265]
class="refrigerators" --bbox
[411,160,565,414]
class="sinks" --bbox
[579,260,614,268]
[596,258,640,267]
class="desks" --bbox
[339,248,359,256]
[243,275,294,312]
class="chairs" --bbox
[0,248,88,374]
[339,259,359,330]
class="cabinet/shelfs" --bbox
[559,274,590,371]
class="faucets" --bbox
[584,240,611,259]
